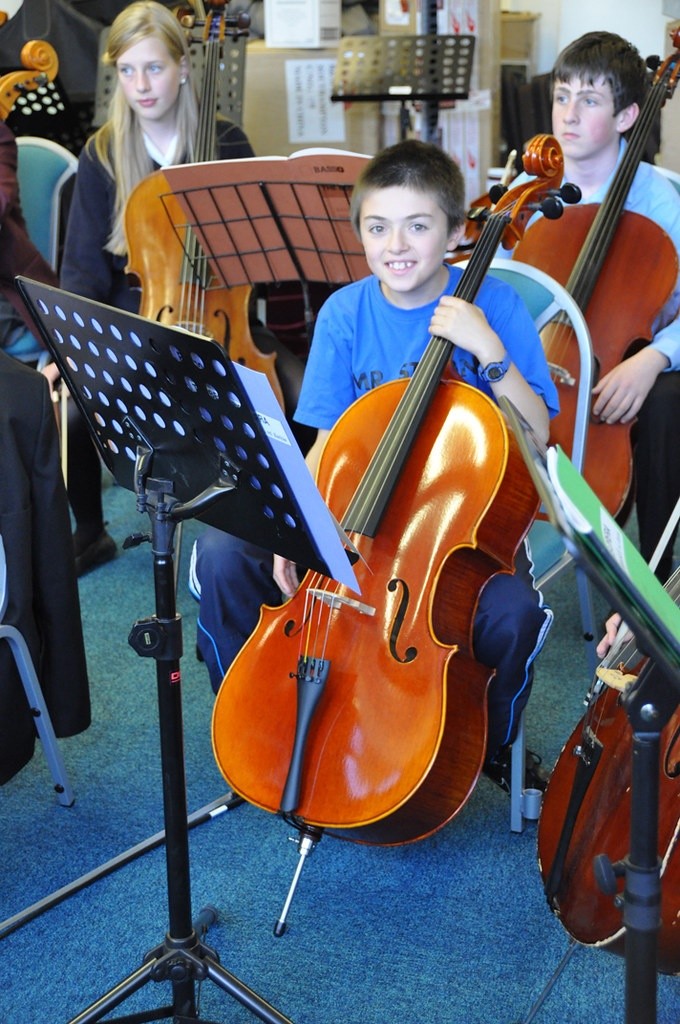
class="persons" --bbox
[0,1,680,798]
[187,139,559,797]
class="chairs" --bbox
[440,257,595,834]
[0,529,73,804]
[10,134,81,372]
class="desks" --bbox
[494,10,544,163]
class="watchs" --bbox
[477,350,512,385]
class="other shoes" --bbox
[480,745,548,797]
[72,520,117,578]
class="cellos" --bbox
[0,39,60,126]
[123,0,286,602]
[210,132,583,938]
[525,563,679,1024]
[510,18,680,524]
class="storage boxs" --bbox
[243,35,420,155]
[263,0,342,50]
